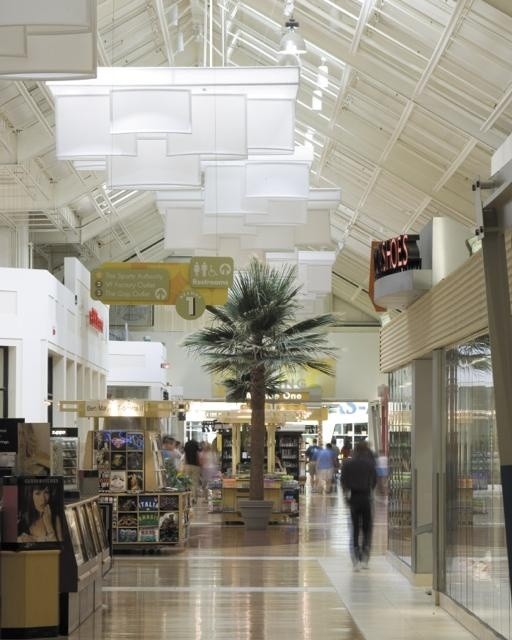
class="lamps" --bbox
[277,0,306,55]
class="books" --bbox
[16,416,188,566]
[220,425,301,517]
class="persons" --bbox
[304,437,390,574]
[166,435,221,505]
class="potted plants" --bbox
[177,254,344,533]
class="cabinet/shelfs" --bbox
[301,433,319,462]
[83,429,193,554]
[218,427,306,517]
[219,476,300,526]
[0,418,112,639]
